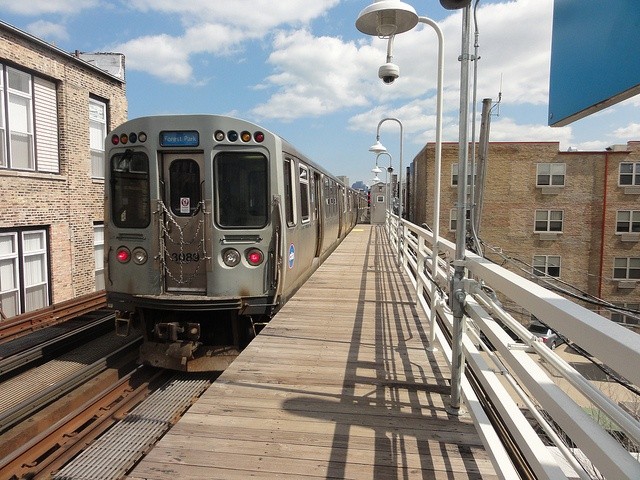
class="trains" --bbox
[101,115,368,372]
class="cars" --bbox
[526,320,569,354]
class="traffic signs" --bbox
[373,174,394,244]
[372,150,395,245]
[379,15,444,351]
[372,165,389,246]
[369,116,405,273]
